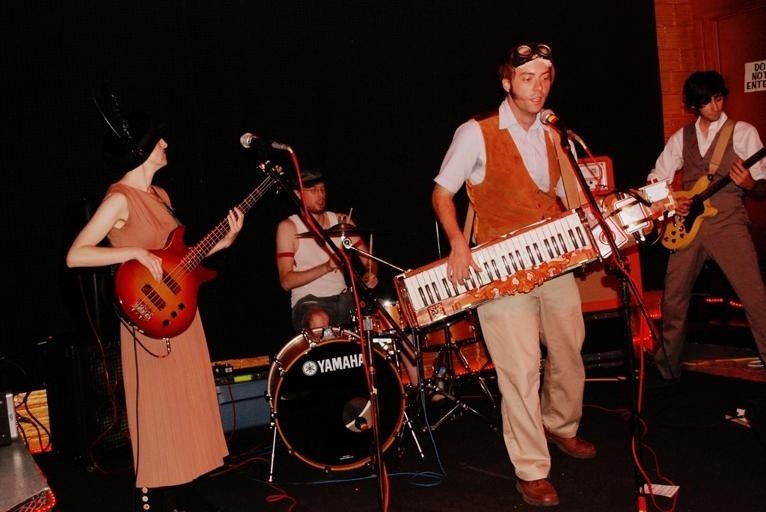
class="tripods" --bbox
[366,222,502,474]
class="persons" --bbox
[65,110,246,491]
[273,171,379,461]
[432,44,599,506]
[648,70,765,406]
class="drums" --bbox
[266,326,406,474]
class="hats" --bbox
[108,107,163,172]
[287,167,328,193]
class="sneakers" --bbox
[644,360,683,385]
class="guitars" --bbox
[656,148,765,248]
[115,163,284,340]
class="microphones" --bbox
[540,109,571,134]
[239,133,285,151]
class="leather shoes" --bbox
[543,427,597,461]
[515,473,561,508]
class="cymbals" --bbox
[295,223,363,238]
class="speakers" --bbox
[580,309,627,371]
[563,193,645,311]
[575,156,615,195]
[42,331,130,468]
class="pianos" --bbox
[395,182,678,331]
